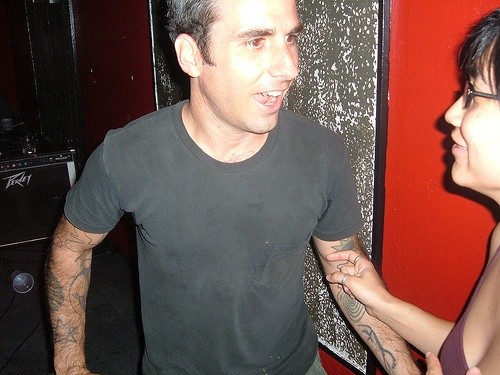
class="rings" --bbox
[354,256,360,262]
[342,275,348,283]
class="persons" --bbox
[325,5,500,375]
[45,0,423,375]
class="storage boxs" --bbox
[0,135,78,248]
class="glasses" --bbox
[460,79,499,109]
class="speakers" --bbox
[0,141,78,247]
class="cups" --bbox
[11,270,35,294]
[20,136,37,154]
[2,119,12,131]
